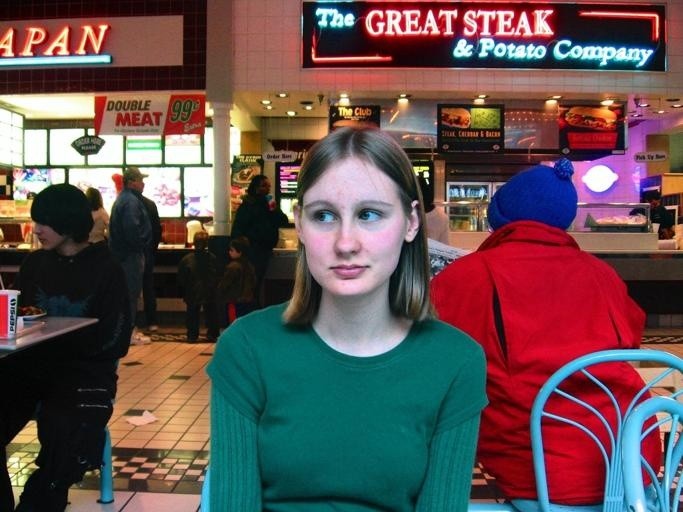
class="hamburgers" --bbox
[440,108,473,129]
[565,106,617,130]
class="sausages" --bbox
[568,131,617,143]
[569,142,617,149]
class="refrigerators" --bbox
[445,181,492,232]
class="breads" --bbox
[596,213,647,225]
[331,119,377,131]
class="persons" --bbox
[415,177,450,246]
[0,182,130,511]
[85,187,110,246]
[111,168,152,346]
[629,190,676,240]
[218,236,254,328]
[204,125,489,510]
[177,231,219,342]
[428,158,662,505]
[120,180,160,331]
[231,176,287,316]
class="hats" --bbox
[122,167,149,182]
[486,156,579,233]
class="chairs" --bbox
[200,458,212,512]
[464,345,683,512]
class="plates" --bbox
[232,171,253,184]
[20,312,49,320]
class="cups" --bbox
[0,289,21,340]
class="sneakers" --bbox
[13,468,74,512]
[129,329,152,346]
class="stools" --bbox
[93,422,118,505]
